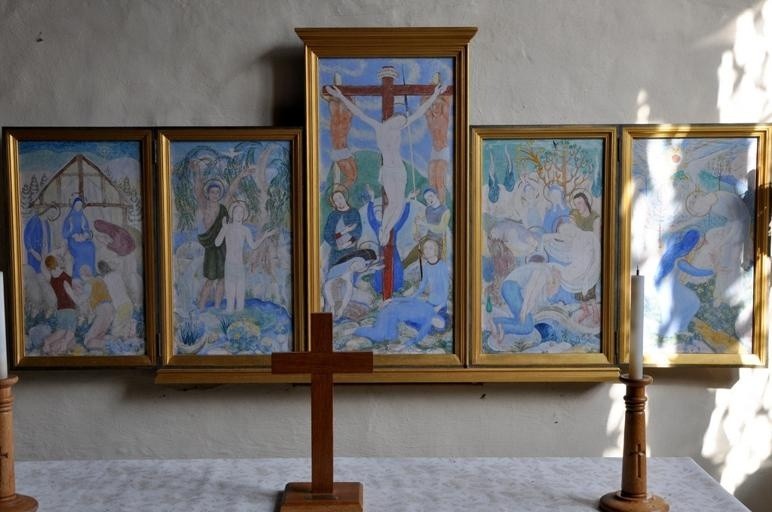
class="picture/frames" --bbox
[158,126,306,370]
[619,123,772,367]
[470,125,617,366]
[0,126,157,368]
[294,27,478,367]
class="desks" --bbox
[13,455,752,512]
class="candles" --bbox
[0,271,8,380]
[630,265,644,378]
